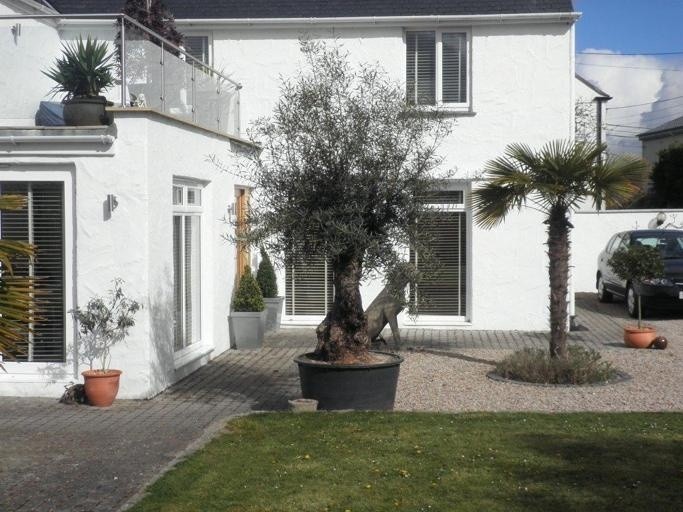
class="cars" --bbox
[595,229,682,318]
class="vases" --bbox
[288,399,318,413]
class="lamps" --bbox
[12,23,21,36]
[656,213,666,224]
[108,194,118,211]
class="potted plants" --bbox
[256,244,284,332]
[67,275,146,406]
[231,264,269,349]
[40,31,122,126]
[201,33,490,416]
[608,242,667,347]
